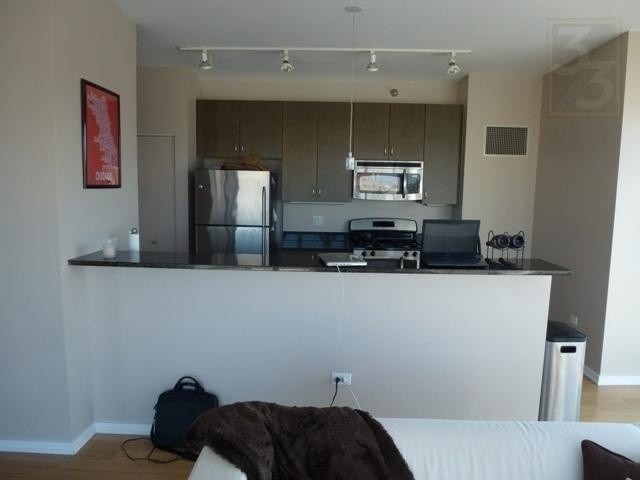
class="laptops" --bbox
[421,219,487,268]
[317,253,367,266]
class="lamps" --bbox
[344,5,363,170]
[198,49,462,77]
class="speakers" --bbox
[487,231,510,249]
[510,234,524,248]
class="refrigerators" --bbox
[194,168,281,253]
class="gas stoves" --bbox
[351,231,420,261]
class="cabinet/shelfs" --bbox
[423,105,463,207]
[283,101,353,203]
[352,103,425,160]
[195,100,283,160]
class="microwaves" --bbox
[353,159,424,203]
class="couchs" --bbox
[187,416,639,480]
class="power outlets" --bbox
[331,373,352,387]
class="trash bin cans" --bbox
[538,319,588,422]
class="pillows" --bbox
[579,438,639,479]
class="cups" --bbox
[101,238,116,259]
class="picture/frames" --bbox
[80,78,121,191]
